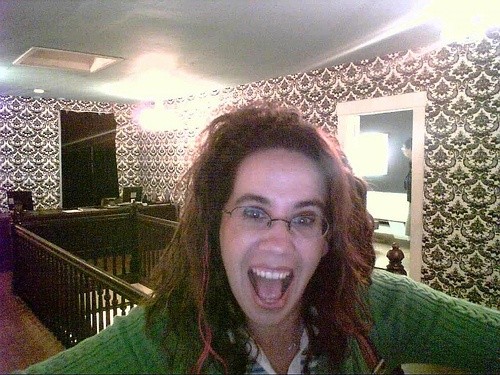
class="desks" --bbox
[0,203,180,271]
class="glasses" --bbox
[218,206,329,241]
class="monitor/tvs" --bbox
[122,187,143,203]
[7,191,33,211]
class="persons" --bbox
[10,103,500,375]
[400,140,411,236]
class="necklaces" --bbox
[273,340,297,361]
